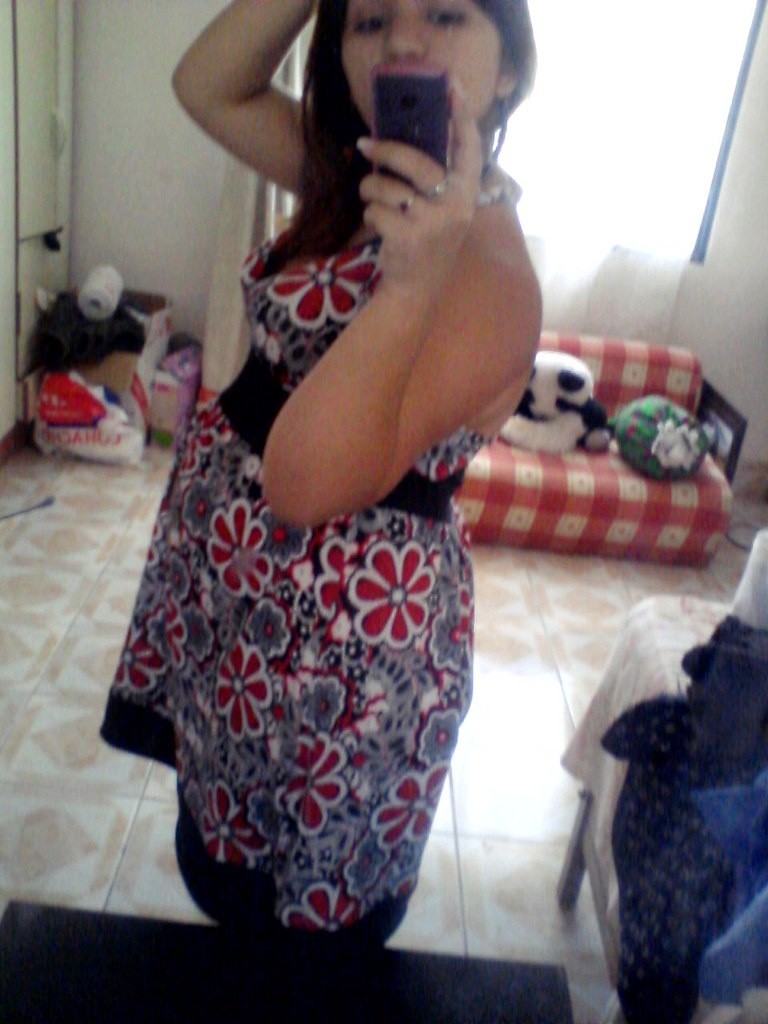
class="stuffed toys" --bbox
[497,350,608,452]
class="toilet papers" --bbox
[77,263,126,322]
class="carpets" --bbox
[1,899,579,1024]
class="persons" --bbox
[99,1,543,954]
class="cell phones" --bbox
[369,62,451,193]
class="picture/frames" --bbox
[698,378,749,484]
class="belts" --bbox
[217,353,468,526]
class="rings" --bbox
[394,190,419,218]
[423,176,450,201]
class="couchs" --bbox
[448,329,734,570]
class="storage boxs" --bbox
[52,283,174,447]
[151,344,200,449]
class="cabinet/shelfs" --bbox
[559,521,768,1024]
[1,0,73,466]
